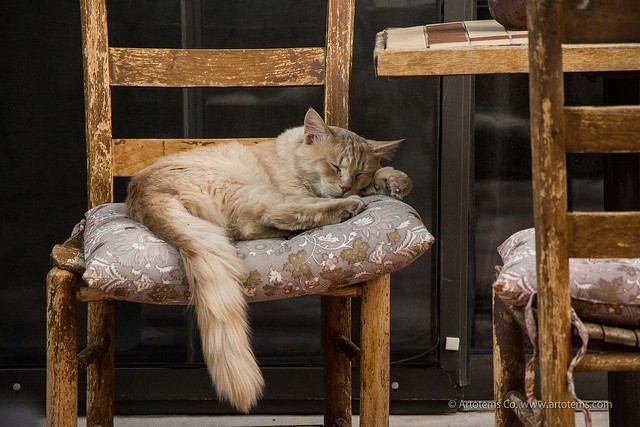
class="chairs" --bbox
[492,0,639,427]
[45,0,391,427]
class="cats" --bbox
[127,108,413,415]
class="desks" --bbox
[373,18,638,427]
